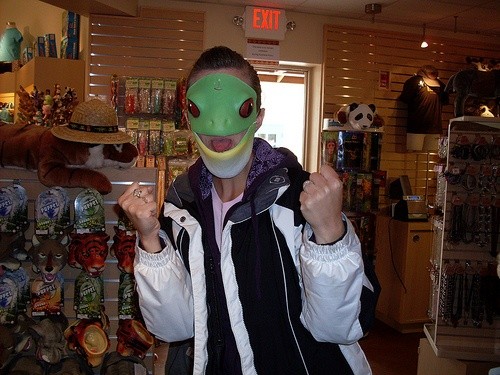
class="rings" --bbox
[303,180,314,190]
[134,189,142,198]
[141,196,149,203]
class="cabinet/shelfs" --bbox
[322,117,500,361]
[112,75,201,199]
[0,166,166,375]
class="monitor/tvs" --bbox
[389,175,412,200]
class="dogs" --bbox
[0,230,69,283]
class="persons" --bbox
[117,43,383,375]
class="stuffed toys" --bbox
[336,101,387,129]
[0,120,138,194]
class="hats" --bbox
[418,65,440,88]
[50,96,136,146]
